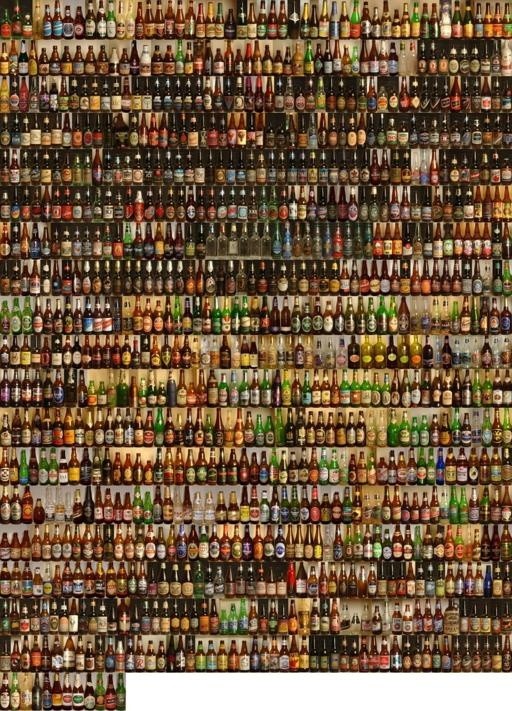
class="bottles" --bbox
[1,2,512,710]
